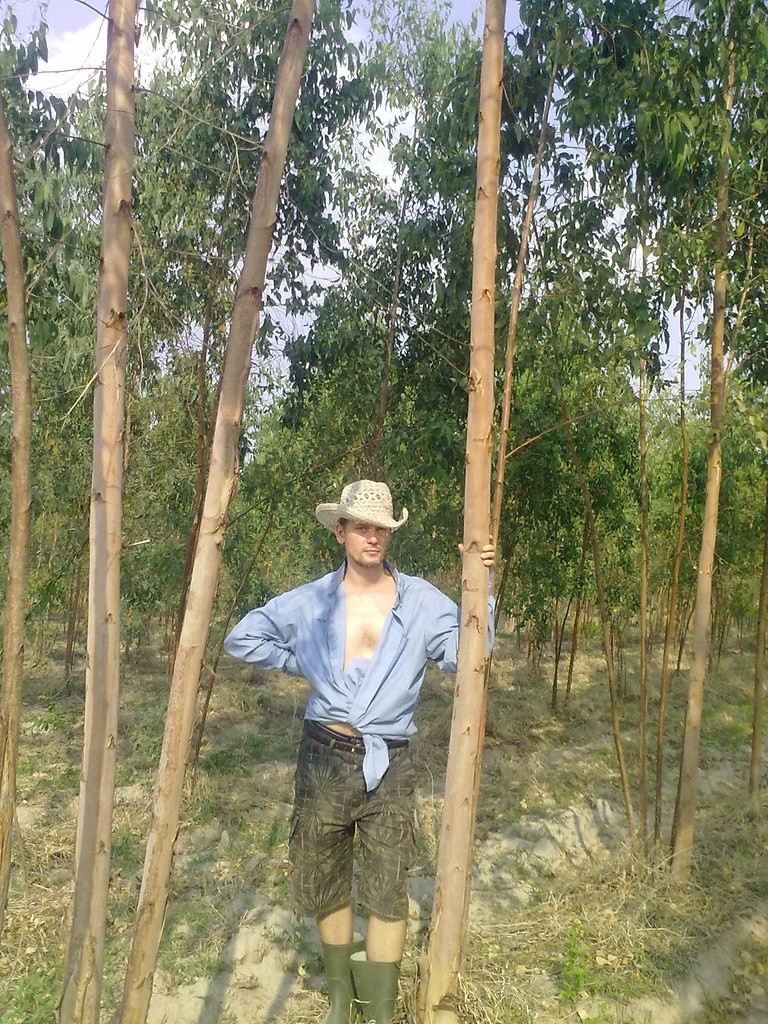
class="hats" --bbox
[315,480,409,534]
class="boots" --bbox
[321,933,401,1024]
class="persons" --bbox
[223,480,496,1024]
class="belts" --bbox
[302,720,409,756]
